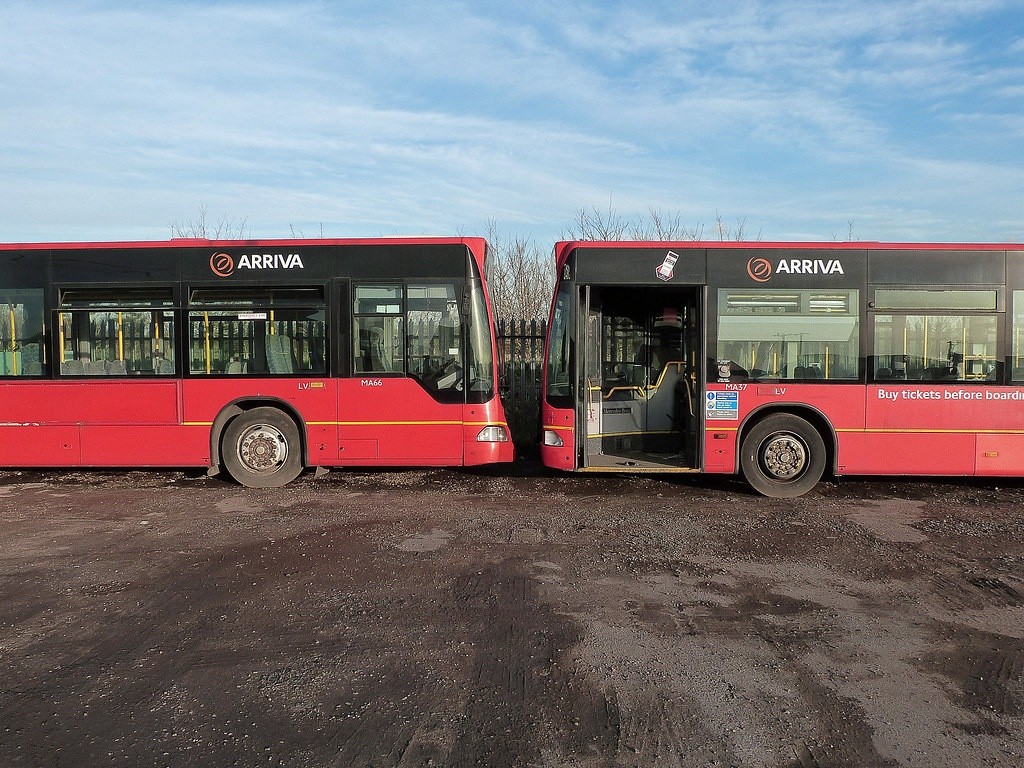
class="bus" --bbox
[538,239,1024,500]
[0,237,516,488]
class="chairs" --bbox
[24,361,127,375]
[723,341,777,378]
[782,365,823,379]
[265,335,301,374]
[875,368,953,381]
[310,337,325,372]
[155,360,174,374]
[224,362,247,374]
[370,326,393,372]
